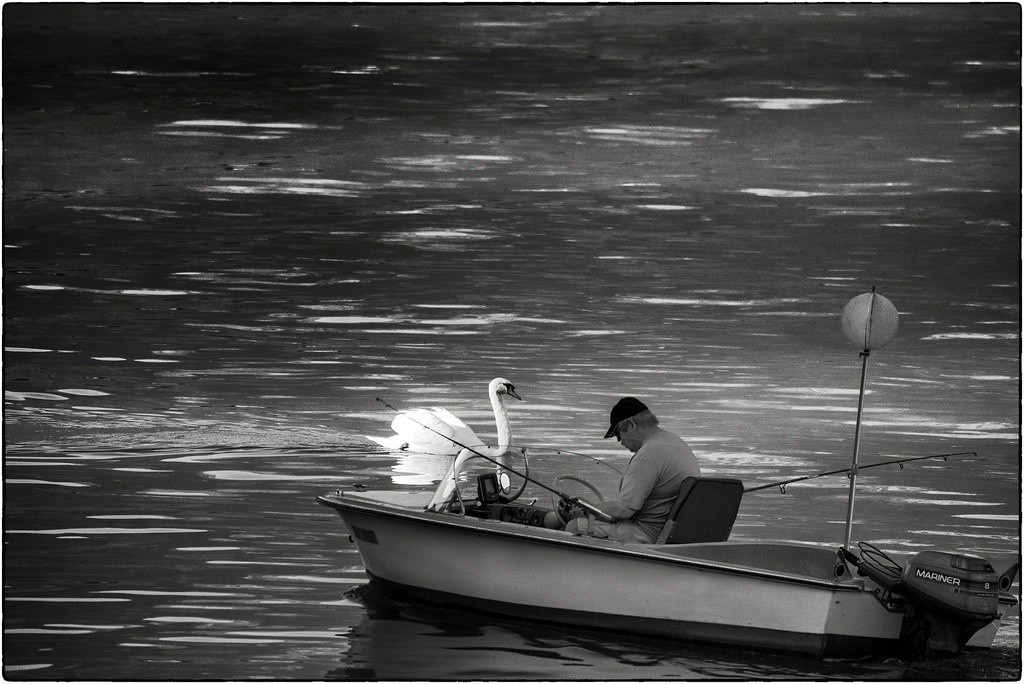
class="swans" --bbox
[364,377,521,457]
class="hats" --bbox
[604,397,648,438]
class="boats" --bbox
[315,444,1020,662]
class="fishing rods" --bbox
[358,385,616,524]
[748,448,979,496]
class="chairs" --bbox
[654,476,744,544]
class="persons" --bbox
[544,397,701,544]
